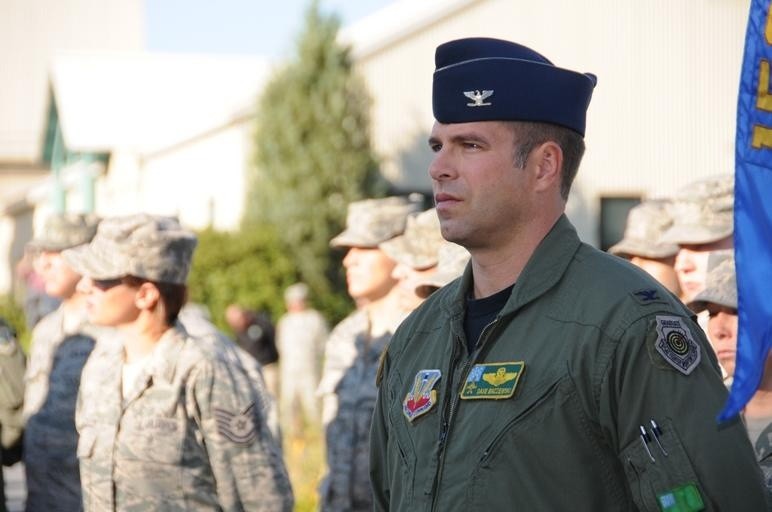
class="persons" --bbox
[367,36,767,510]
[226,193,472,511]
[608,178,771,511]
[0,210,296,512]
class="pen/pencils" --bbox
[639,424,656,463]
[649,418,668,457]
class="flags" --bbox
[722,3,772,413]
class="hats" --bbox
[23,203,100,255]
[432,37,598,137]
[607,173,739,314]
[330,192,467,302]
[59,213,199,285]
